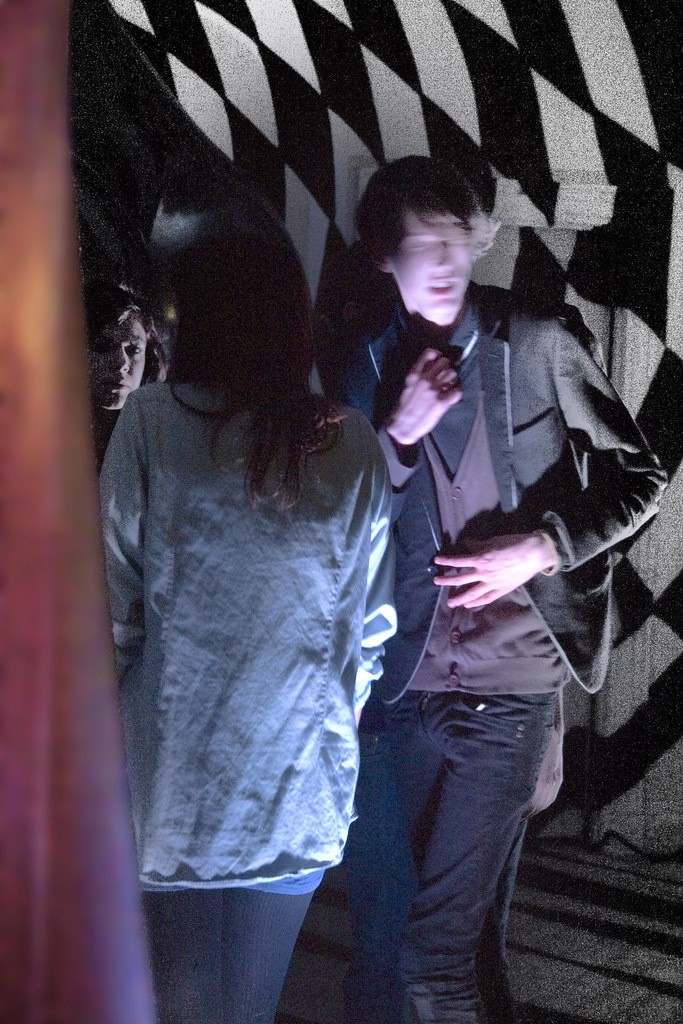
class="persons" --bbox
[473,689,565,1024]
[331,155,666,1024]
[82,281,167,473]
[97,240,399,1024]
